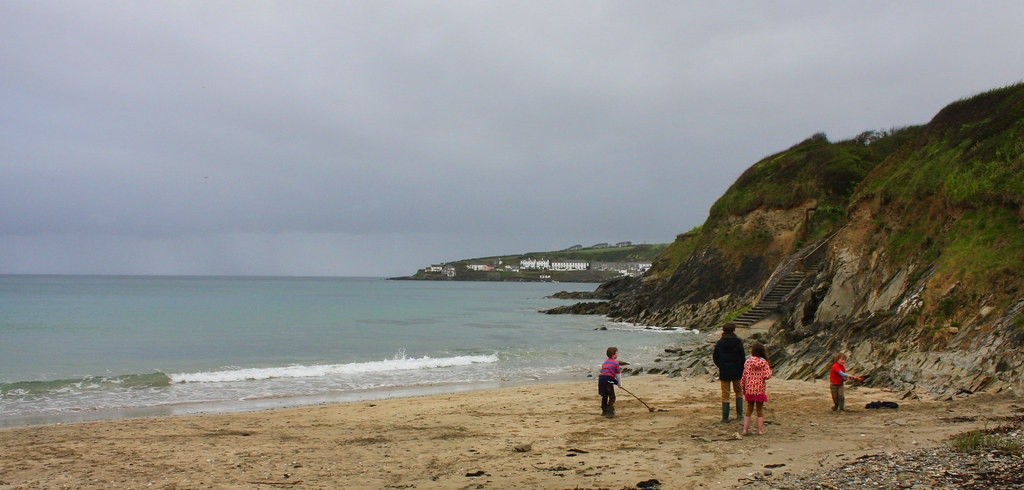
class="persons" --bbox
[829,352,863,415]
[713,323,746,424]
[598,347,622,417]
[739,341,773,436]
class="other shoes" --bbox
[832,405,838,411]
[839,410,845,415]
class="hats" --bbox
[723,323,735,332]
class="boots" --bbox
[736,398,744,419]
[757,417,766,434]
[721,402,731,423]
[743,417,750,434]
[606,406,615,418]
[601,405,608,416]
